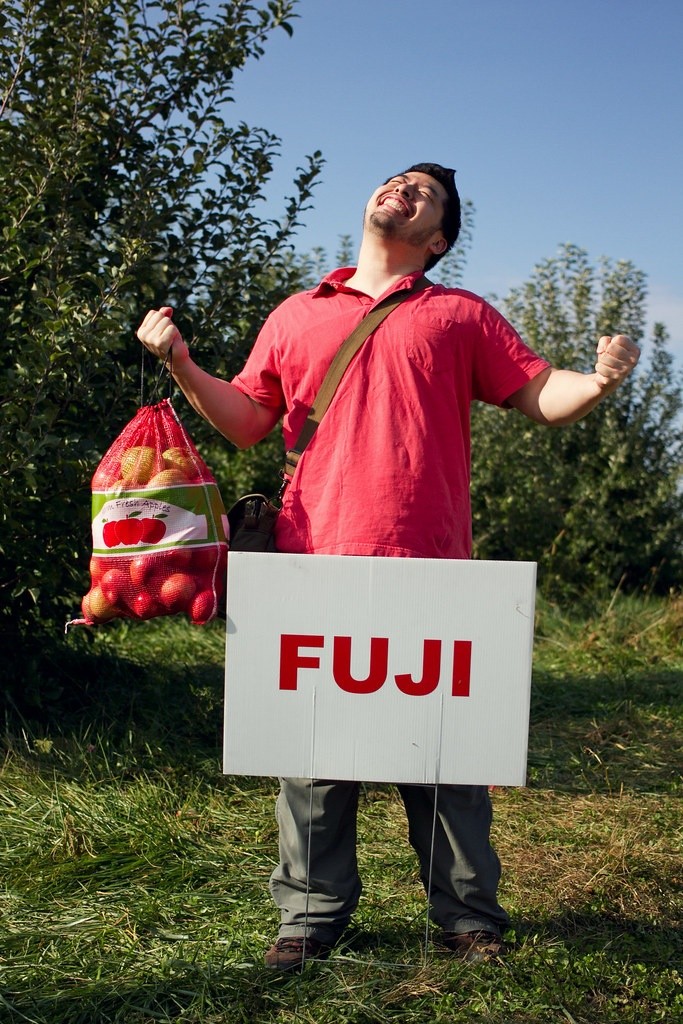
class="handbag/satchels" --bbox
[216,494,279,618]
[62,344,231,634]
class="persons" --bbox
[135,163,641,968]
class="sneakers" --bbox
[265,936,322,971]
[437,931,508,963]
